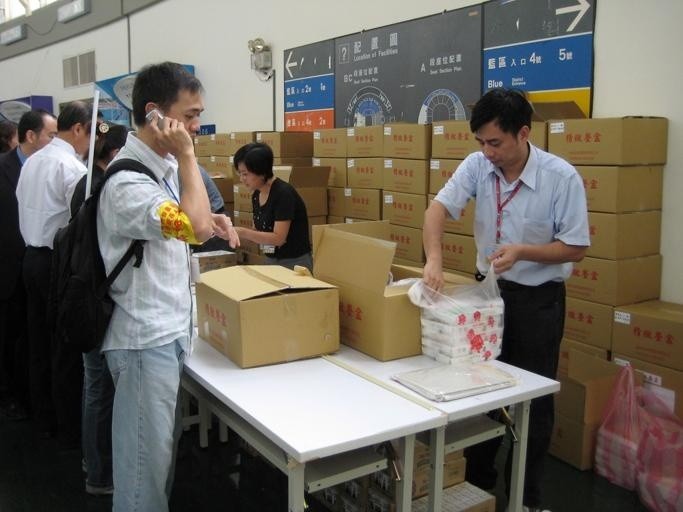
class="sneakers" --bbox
[84,477,113,496]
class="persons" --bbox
[231,142,312,273]
[423,88,591,512]
[96,60,241,512]
[0,100,227,492]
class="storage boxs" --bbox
[548,336,611,471]
[563,296,659,353]
[195,265,339,370]
[612,299,682,372]
[309,220,477,361]
[311,119,546,237]
[190,129,330,279]
[579,211,661,260]
[311,444,497,512]
[547,115,669,166]
[574,164,663,213]
[611,354,683,424]
[563,255,662,307]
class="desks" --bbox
[177,282,561,512]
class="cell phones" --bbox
[144,108,164,129]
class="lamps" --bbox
[57,0,91,24]
[248,38,266,54]
[0,24,27,46]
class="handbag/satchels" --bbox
[43,199,115,366]
